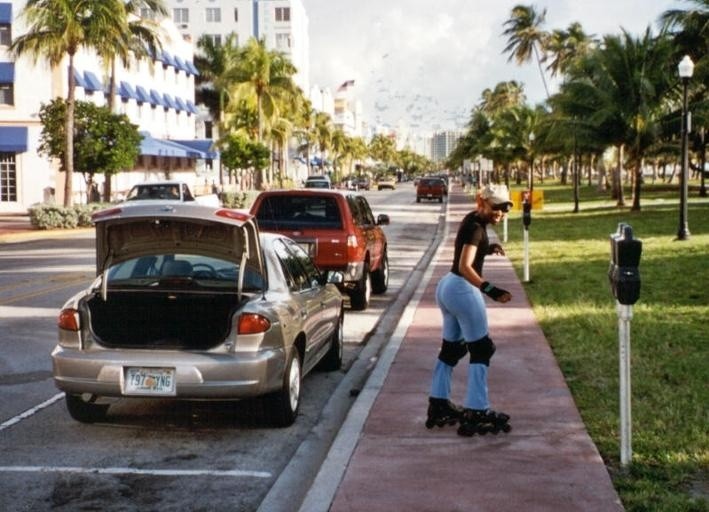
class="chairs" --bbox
[159,259,194,277]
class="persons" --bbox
[424,183,514,426]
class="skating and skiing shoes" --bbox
[457,405,512,439]
[424,396,464,429]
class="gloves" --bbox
[479,281,513,304]
[487,241,505,257]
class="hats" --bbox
[478,185,514,207]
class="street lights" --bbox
[529,132,535,191]
[677,55,695,240]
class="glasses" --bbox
[487,201,512,213]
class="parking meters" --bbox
[521,199,532,282]
[607,222,642,465]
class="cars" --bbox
[414,173,449,203]
